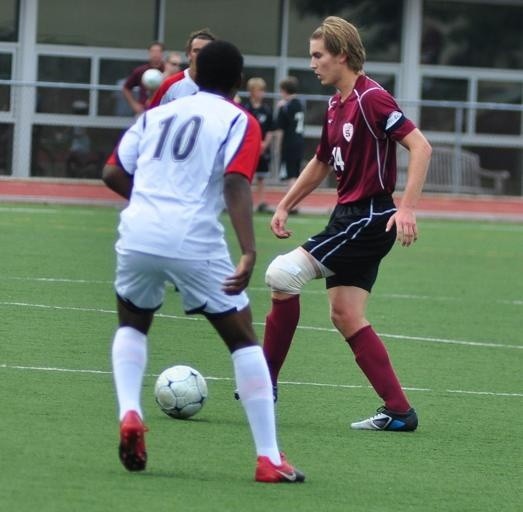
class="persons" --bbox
[234,15,433,432]
[101,39,306,483]
[3,99,92,181]
[120,27,305,216]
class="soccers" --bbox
[141,69,164,89]
[154,365,207,418]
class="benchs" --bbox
[421,145,510,194]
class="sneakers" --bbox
[119,412,147,471]
[350,408,418,432]
[255,453,304,483]
